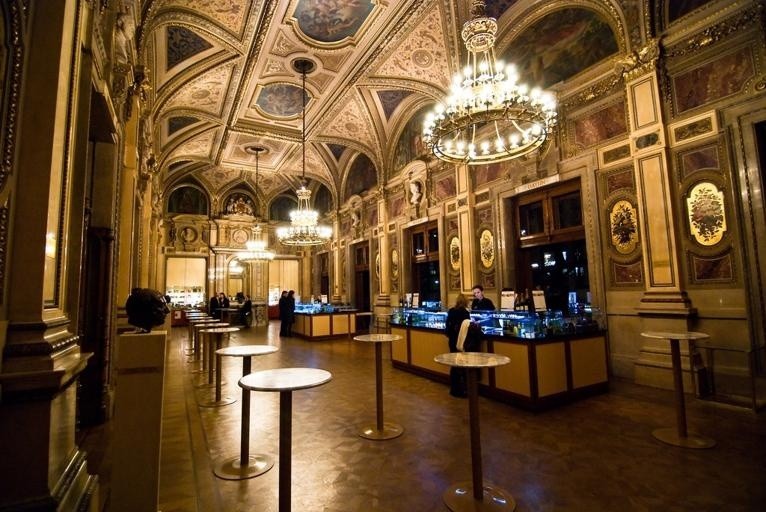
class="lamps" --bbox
[273,60,331,247]
[422,0,558,166]
[236,147,273,265]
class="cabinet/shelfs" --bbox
[389,307,611,413]
[285,304,357,341]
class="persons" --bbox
[219,292,229,308]
[285,290,295,336]
[238,294,251,321]
[446,294,471,398]
[279,291,288,337]
[126,286,169,333]
[470,284,496,310]
[209,292,221,319]
